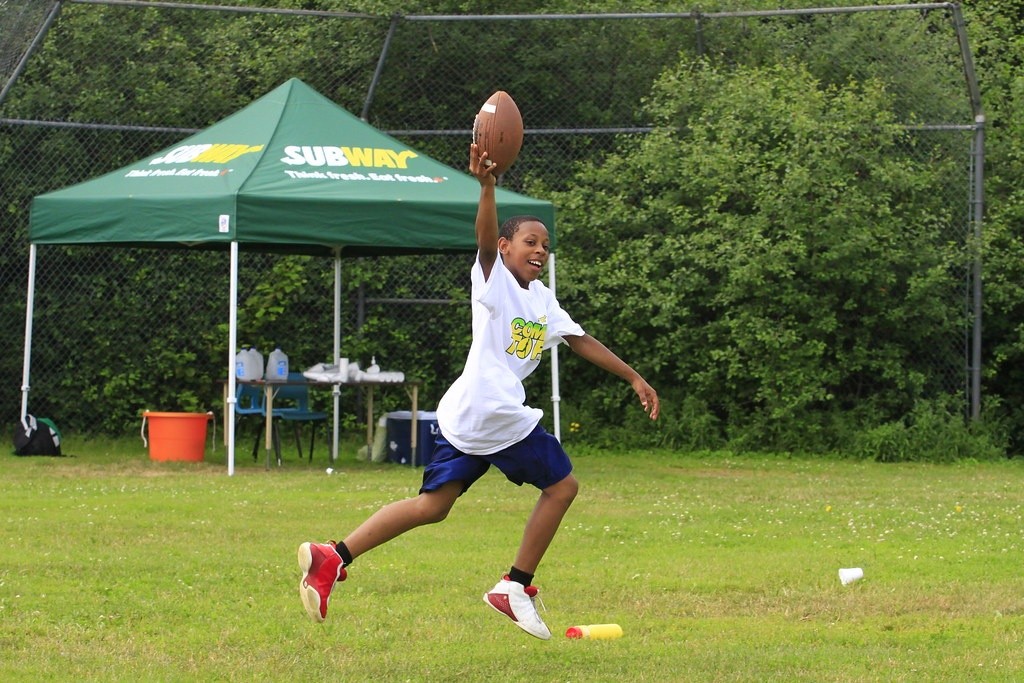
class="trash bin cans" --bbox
[143,411,214,463]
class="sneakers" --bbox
[482,571,552,641]
[298,540,347,624]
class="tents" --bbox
[16,77,563,478]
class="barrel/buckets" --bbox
[140,410,215,462]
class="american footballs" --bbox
[471,90,525,178]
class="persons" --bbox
[297,144,659,642]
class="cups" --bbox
[839,568,863,587]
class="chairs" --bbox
[252,373,335,466]
[232,375,303,464]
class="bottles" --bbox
[266,347,289,380]
[236,348,253,381]
[248,346,264,379]
[566,624,623,641]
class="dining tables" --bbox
[290,373,407,464]
